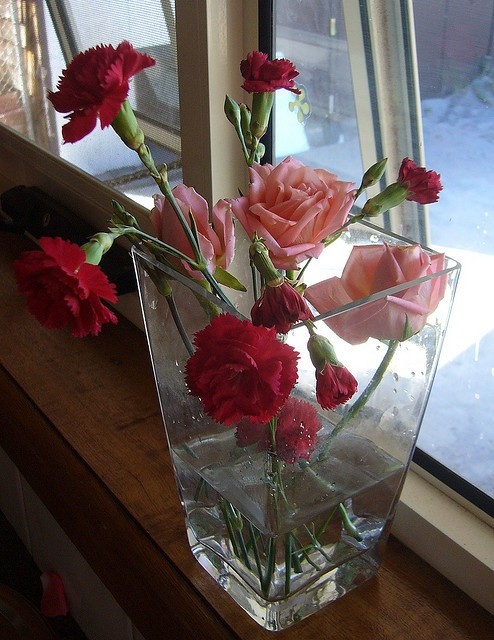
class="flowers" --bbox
[9,37,446,599]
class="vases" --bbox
[127,215,462,632]
